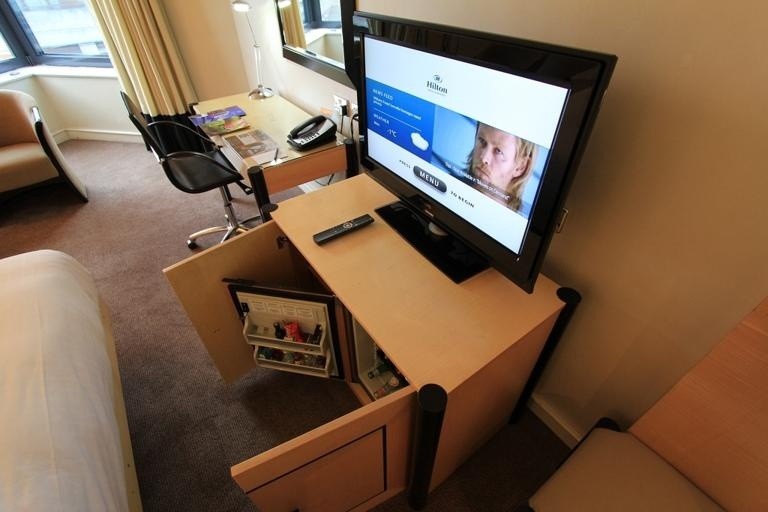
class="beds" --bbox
[1,248,145,512]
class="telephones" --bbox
[287,115,337,151]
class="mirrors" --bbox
[274,0,361,93]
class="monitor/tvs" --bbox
[352,10,619,294]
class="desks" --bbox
[190,88,359,223]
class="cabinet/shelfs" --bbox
[159,172,584,512]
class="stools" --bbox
[523,415,726,512]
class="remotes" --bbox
[313,213,374,245]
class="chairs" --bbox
[1,89,90,204]
[119,90,264,250]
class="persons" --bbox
[457,120,539,212]
[188,108,230,128]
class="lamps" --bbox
[231,0,274,101]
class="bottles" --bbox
[374,374,403,400]
[367,364,390,380]
[272,322,286,340]
[256,346,325,368]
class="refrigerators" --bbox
[219,277,409,402]
